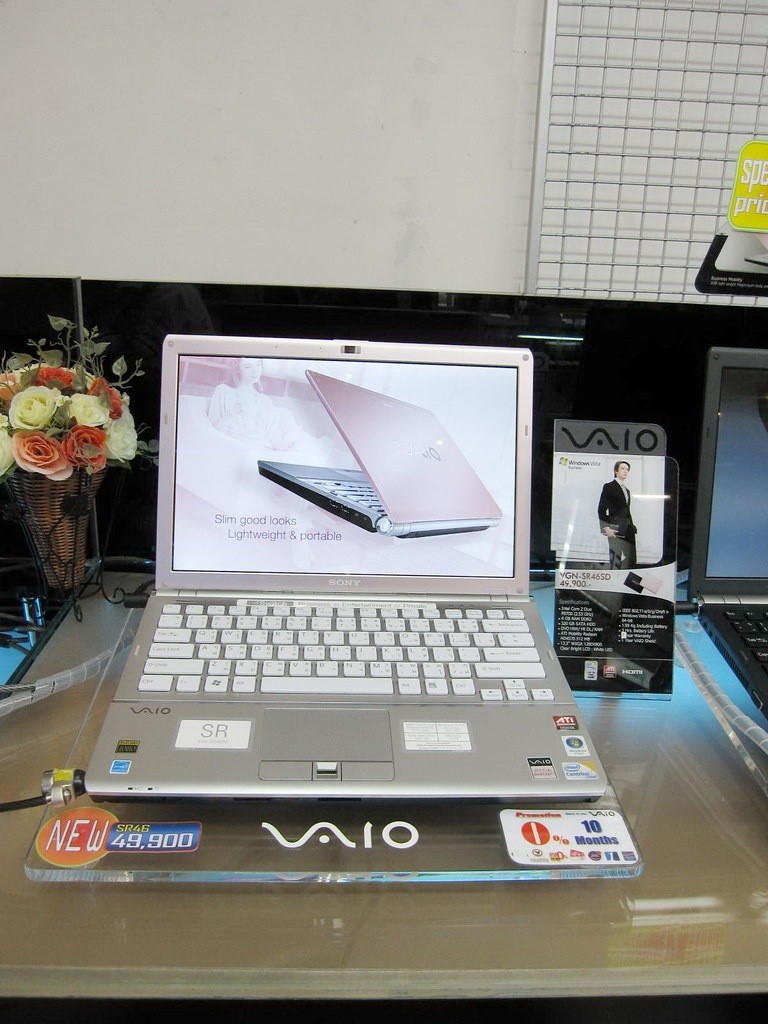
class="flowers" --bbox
[0,315,159,484]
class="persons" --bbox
[597,461,638,570]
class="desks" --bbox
[0,543,768,1024]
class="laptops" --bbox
[257,369,504,539]
[87,334,608,807]
[690,345,768,722]
